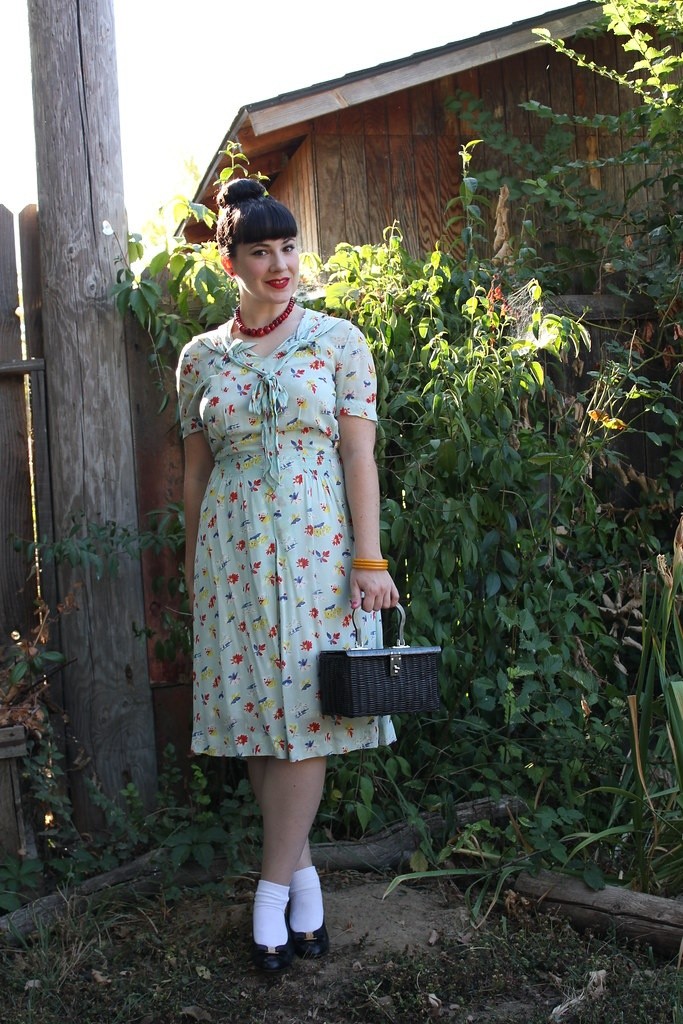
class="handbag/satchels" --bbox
[319,599,440,718]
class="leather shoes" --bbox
[250,920,329,975]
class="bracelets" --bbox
[350,558,389,572]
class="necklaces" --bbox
[232,295,295,338]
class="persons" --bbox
[176,177,402,983]
